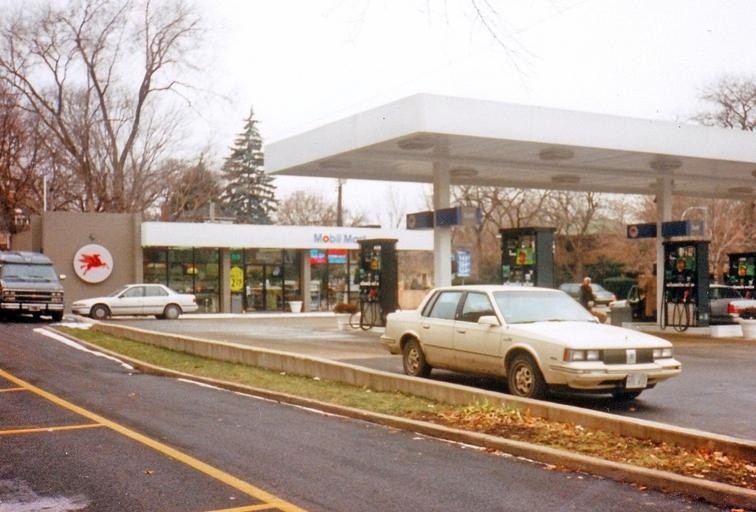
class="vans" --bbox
[0,249,64,322]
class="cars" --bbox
[71,282,199,319]
[379,283,681,398]
[559,281,615,306]
[626,284,756,327]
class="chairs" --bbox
[437,300,455,318]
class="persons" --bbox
[678,286,692,304]
[517,250,531,266]
[676,256,691,270]
[739,264,749,276]
[577,271,616,313]
